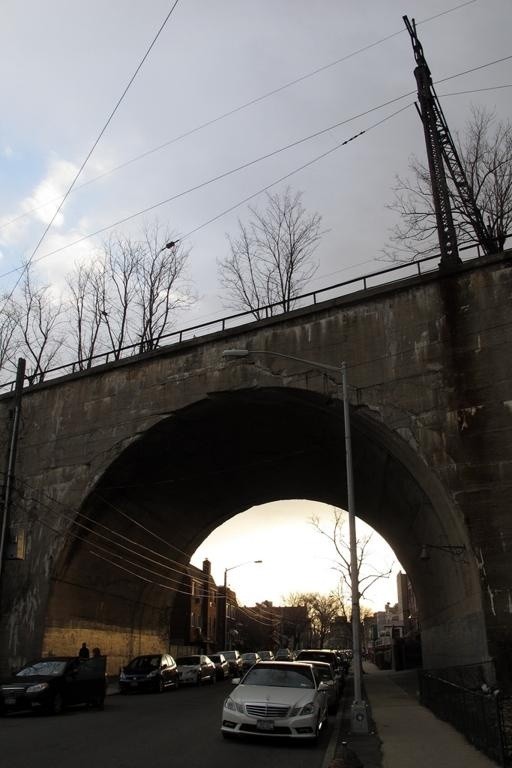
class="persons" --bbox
[79,642,89,658]
[90,648,107,712]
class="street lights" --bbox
[221,348,369,734]
[224,559,263,650]
